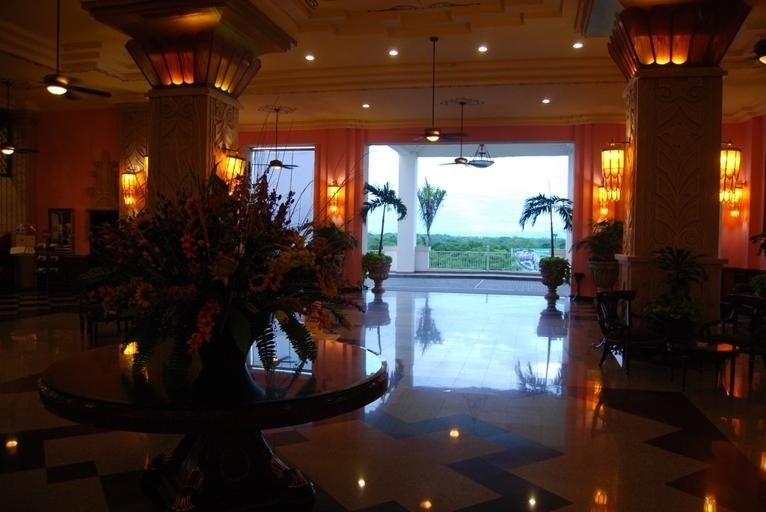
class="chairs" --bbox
[594,290,665,376]
[695,292,765,400]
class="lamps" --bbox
[46,85,68,97]
[600,136,631,179]
[425,134,440,142]
[720,137,743,192]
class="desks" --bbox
[35,344,390,511]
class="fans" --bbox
[409,35,468,143]
[8,0,115,102]
[253,106,298,171]
[441,103,473,166]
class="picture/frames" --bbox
[48,208,75,254]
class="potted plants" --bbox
[568,220,623,318]
[361,251,393,294]
[640,245,708,339]
[538,255,573,314]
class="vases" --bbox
[194,310,267,400]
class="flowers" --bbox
[44,110,381,379]
